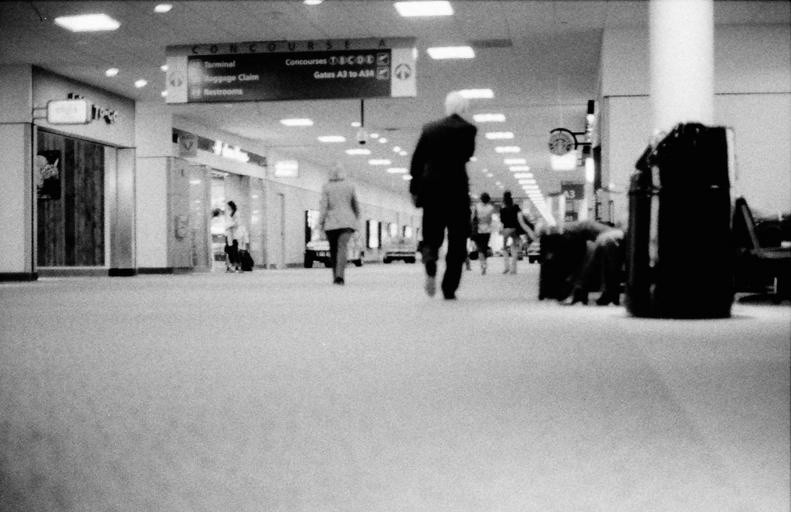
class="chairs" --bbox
[737,198,791,303]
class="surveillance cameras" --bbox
[356,128,368,144]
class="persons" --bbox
[209,207,230,262]
[408,90,478,300]
[224,200,242,272]
[466,190,626,305]
[316,165,361,284]
